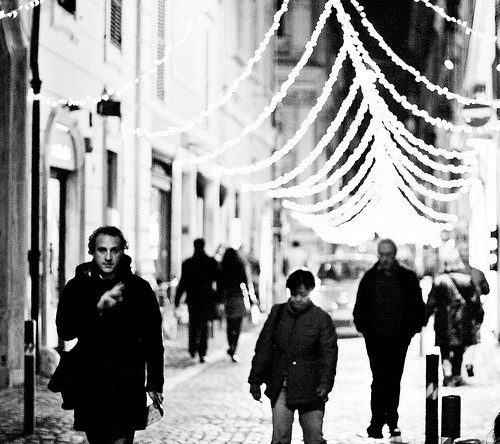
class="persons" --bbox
[282,240,309,273]
[427,244,484,388]
[247,269,339,444]
[174,236,219,364]
[211,246,260,364]
[352,238,427,444]
[463,261,490,377]
[56,224,166,444]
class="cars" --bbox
[311,252,387,332]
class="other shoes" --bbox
[466,366,474,377]
[443,379,463,386]
[367,427,384,439]
[227,349,239,362]
[191,355,195,357]
[388,427,401,436]
[200,359,207,363]
